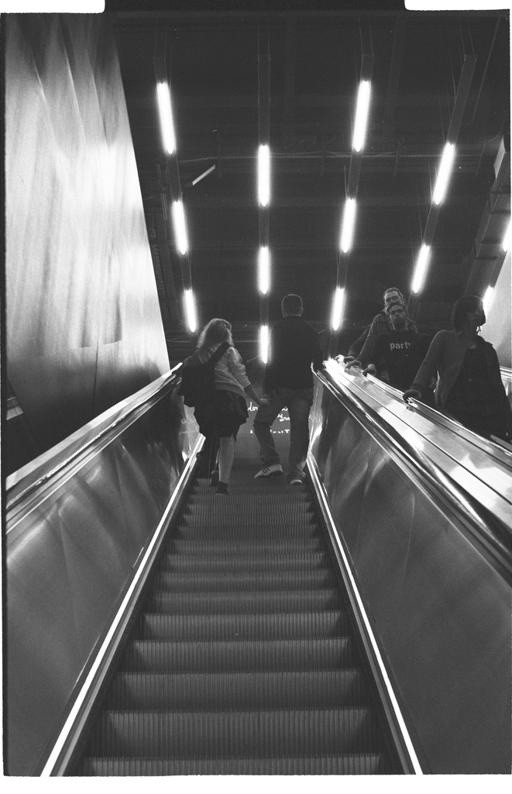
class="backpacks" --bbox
[179,341,230,407]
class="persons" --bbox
[362,302,437,408]
[253,294,327,484]
[344,323,369,363]
[403,296,512,442]
[346,287,417,367]
[194,318,271,494]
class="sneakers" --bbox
[254,463,283,479]
[216,481,230,495]
[210,470,219,485]
[290,479,302,486]
